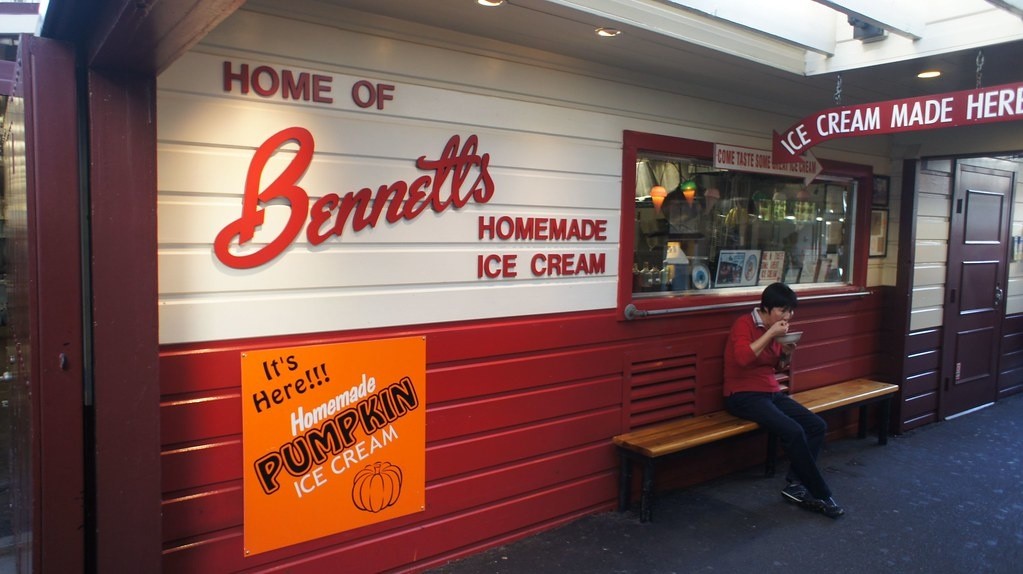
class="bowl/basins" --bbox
[774,331,803,345]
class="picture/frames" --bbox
[868,210,889,257]
[872,174,890,209]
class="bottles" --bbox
[640,261,651,292]
[633,263,641,293]
[649,265,662,292]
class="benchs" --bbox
[612,377,899,522]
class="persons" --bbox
[723,282,845,517]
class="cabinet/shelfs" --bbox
[631,158,850,291]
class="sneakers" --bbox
[781,483,814,504]
[802,497,846,517]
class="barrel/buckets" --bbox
[663,242,689,291]
